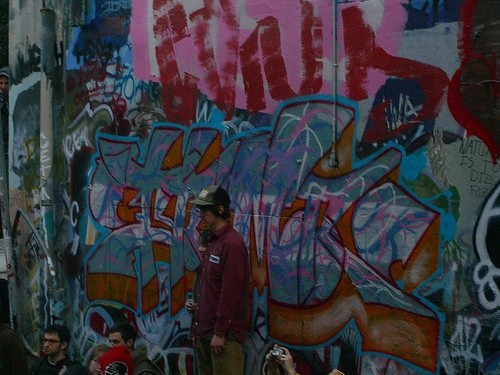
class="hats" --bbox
[188,185,230,210]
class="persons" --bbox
[61,322,158,375]
[31,324,72,375]
[185,185,252,375]
[0,317,32,375]
[262,344,346,375]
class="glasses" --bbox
[42,338,60,344]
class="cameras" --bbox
[271,347,285,362]
